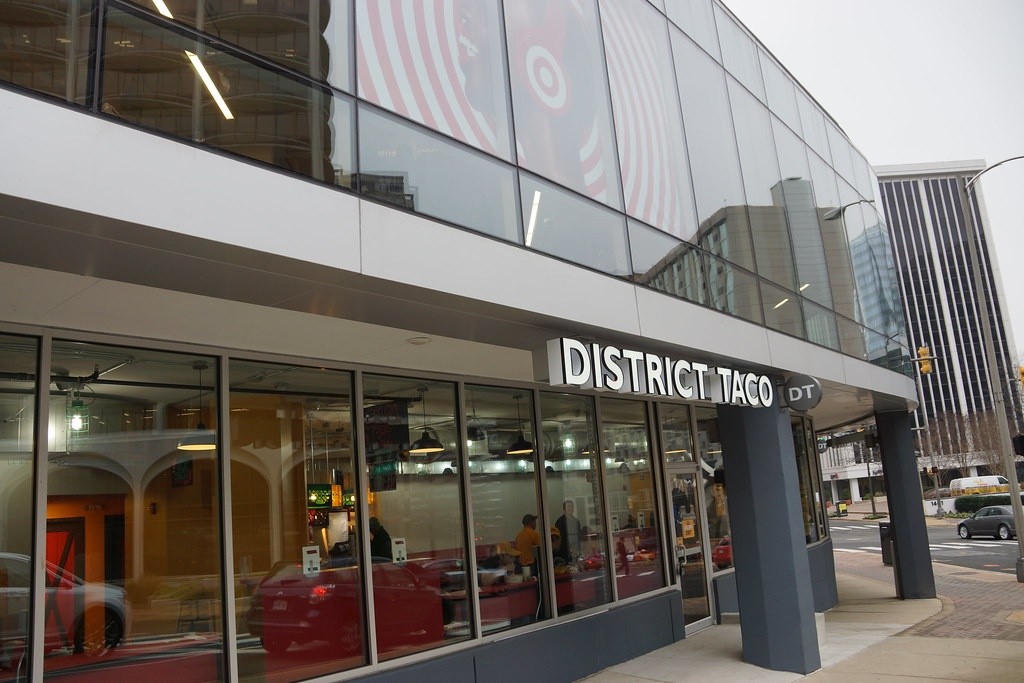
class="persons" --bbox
[514,514,561,576]
[616,537,631,576]
[369,517,393,561]
[555,500,583,562]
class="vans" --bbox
[949,475,1022,493]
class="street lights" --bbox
[962,155,1024,586]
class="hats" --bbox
[369,517,379,524]
[550,526,561,537]
[496,542,522,556]
[522,514,538,525]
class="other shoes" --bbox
[626,574,633,577]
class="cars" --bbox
[577,558,602,572]
[626,550,656,562]
[392,557,514,597]
[0,551,133,663]
[956,504,1024,539]
[712,536,732,569]
[245,553,445,656]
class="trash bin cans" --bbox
[836,501,847,517]
[878,520,893,564]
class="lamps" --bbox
[409,387,444,453]
[582,441,609,455]
[665,438,687,453]
[177,361,216,450]
[507,395,533,454]
[63,380,90,453]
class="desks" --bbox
[441,554,662,623]
[173,592,216,633]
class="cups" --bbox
[522,567,530,581]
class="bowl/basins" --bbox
[505,577,521,583]
[481,573,499,586]
